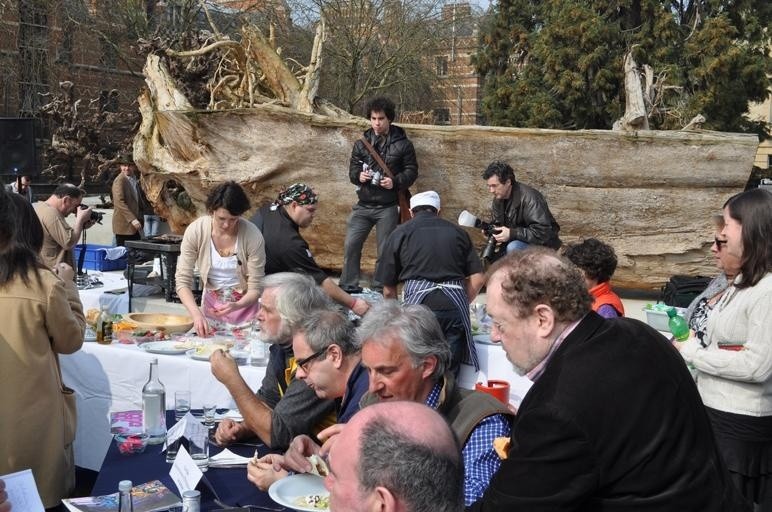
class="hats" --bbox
[410,191,440,211]
[116,154,135,166]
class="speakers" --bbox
[0,117,43,177]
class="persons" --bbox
[9,176,32,206]
[339,97,420,293]
[481,161,563,264]
[323,401,465,512]
[0,478,13,512]
[175,180,265,338]
[671,188,771,511]
[375,190,488,382]
[463,246,755,512]
[247,311,369,493]
[0,190,87,512]
[210,273,341,455]
[33,183,96,271]
[112,156,146,246]
[681,214,743,347]
[353,299,516,507]
[560,238,624,319]
[248,182,372,317]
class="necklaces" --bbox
[212,220,239,258]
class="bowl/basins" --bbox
[115,434,149,454]
[126,312,194,336]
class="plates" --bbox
[140,340,193,355]
[187,345,228,358]
[474,335,499,345]
[268,474,330,511]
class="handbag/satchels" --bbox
[361,136,412,224]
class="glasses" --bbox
[297,346,327,368]
[715,237,727,251]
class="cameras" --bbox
[475,217,500,260]
[367,169,383,187]
[75,204,105,225]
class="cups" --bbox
[165,436,177,463]
[189,437,208,472]
[202,404,214,427]
[175,392,189,420]
[216,330,234,357]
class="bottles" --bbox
[142,358,168,444]
[96,304,113,344]
[182,490,201,512]
[117,480,134,512]
[666,306,695,371]
